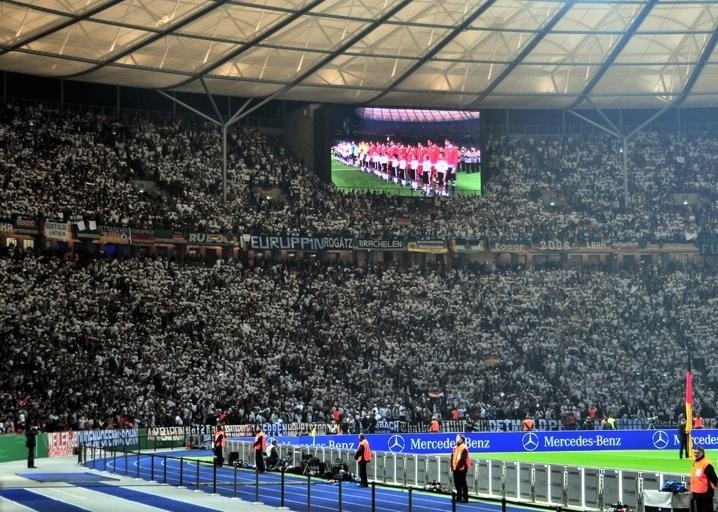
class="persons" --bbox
[25,422,39,468]
[212,424,224,467]
[679,419,689,458]
[450,434,472,504]
[252,425,266,473]
[265,440,284,470]
[690,446,718,512]
[355,433,373,488]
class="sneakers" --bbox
[680,456,689,459]
[255,469,265,473]
[214,463,222,467]
[401,184,427,195]
[357,483,368,488]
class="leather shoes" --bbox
[450,498,468,503]
